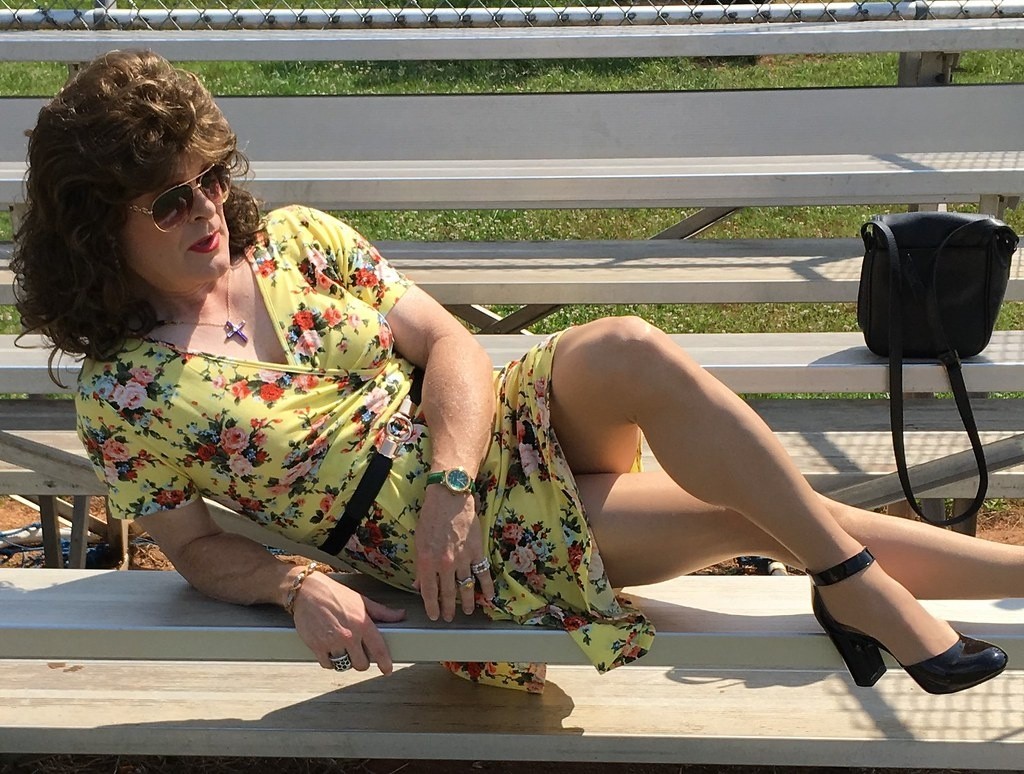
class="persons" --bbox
[11,47,1024,695]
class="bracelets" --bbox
[284,562,318,614]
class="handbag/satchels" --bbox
[856,213,1020,525]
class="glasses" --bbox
[117,162,231,233]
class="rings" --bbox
[457,575,475,589]
[330,654,351,672]
[471,557,491,575]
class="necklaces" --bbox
[157,268,248,343]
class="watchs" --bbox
[424,467,477,496]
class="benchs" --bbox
[0,150,1024,770]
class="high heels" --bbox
[804,545,1009,694]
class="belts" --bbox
[316,368,426,557]
